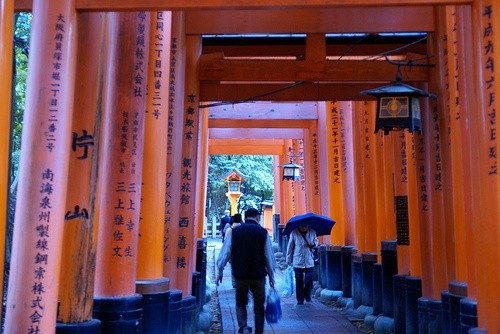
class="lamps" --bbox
[359,56,438,135]
[280,152,305,181]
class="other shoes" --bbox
[297,300,304,306]
[306,300,312,306]
[239,326,249,334]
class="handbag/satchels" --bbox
[266,284,281,323]
[313,251,319,267]
[281,264,294,298]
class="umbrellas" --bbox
[282,213,335,242]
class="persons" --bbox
[215,208,277,334]
[286,221,318,305]
[219,211,243,288]
